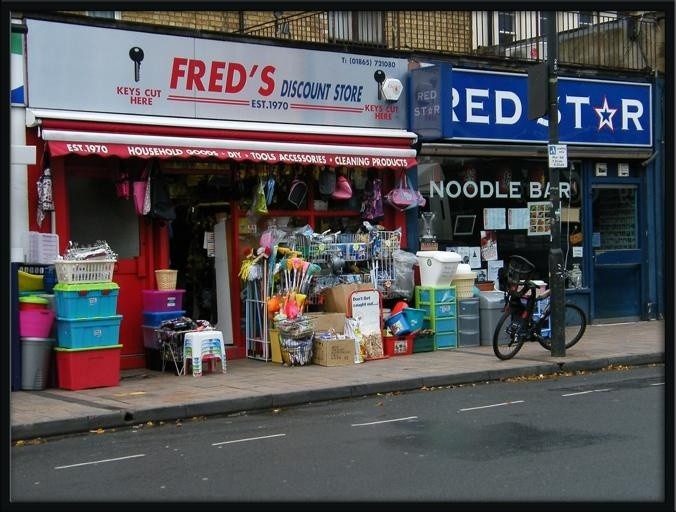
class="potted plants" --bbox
[476,279,496,294]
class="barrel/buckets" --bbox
[19,336,57,391]
[383,311,411,337]
[402,307,427,332]
[20,294,48,315]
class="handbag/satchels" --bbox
[133,174,176,220]
[256,185,268,215]
[331,175,352,199]
[362,177,384,219]
[392,189,426,207]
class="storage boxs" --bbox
[516,276,552,300]
[381,334,414,356]
[303,310,346,334]
[411,328,436,354]
[418,236,438,251]
[314,332,355,367]
[18,231,186,394]
[444,241,484,270]
[323,280,373,315]
[416,251,462,287]
[268,328,284,364]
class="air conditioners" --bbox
[502,42,548,61]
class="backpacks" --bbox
[319,166,336,194]
[263,173,279,205]
[287,180,308,208]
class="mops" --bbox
[238,244,271,362]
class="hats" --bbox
[452,264,477,279]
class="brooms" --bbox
[269,245,322,332]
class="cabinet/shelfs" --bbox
[414,285,459,355]
[521,295,553,340]
[455,295,483,349]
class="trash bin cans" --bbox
[480,291,513,346]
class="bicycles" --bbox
[492,252,587,363]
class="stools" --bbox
[180,328,228,378]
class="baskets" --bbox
[155,269,178,291]
[28,231,59,263]
[451,279,474,298]
[278,334,315,367]
[55,259,117,285]
[273,315,318,339]
